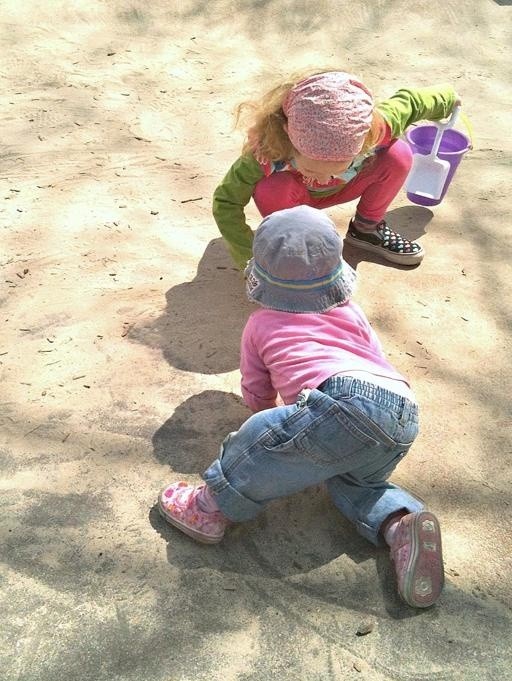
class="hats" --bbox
[243,204,358,314]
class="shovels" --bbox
[403,106,461,201]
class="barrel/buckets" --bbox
[404,110,475,206]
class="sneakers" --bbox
[158,480,224,545]
[388,511,444,608]
[346,217,425,265]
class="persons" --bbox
[212,68,461,273]
[158,204,444,607]
